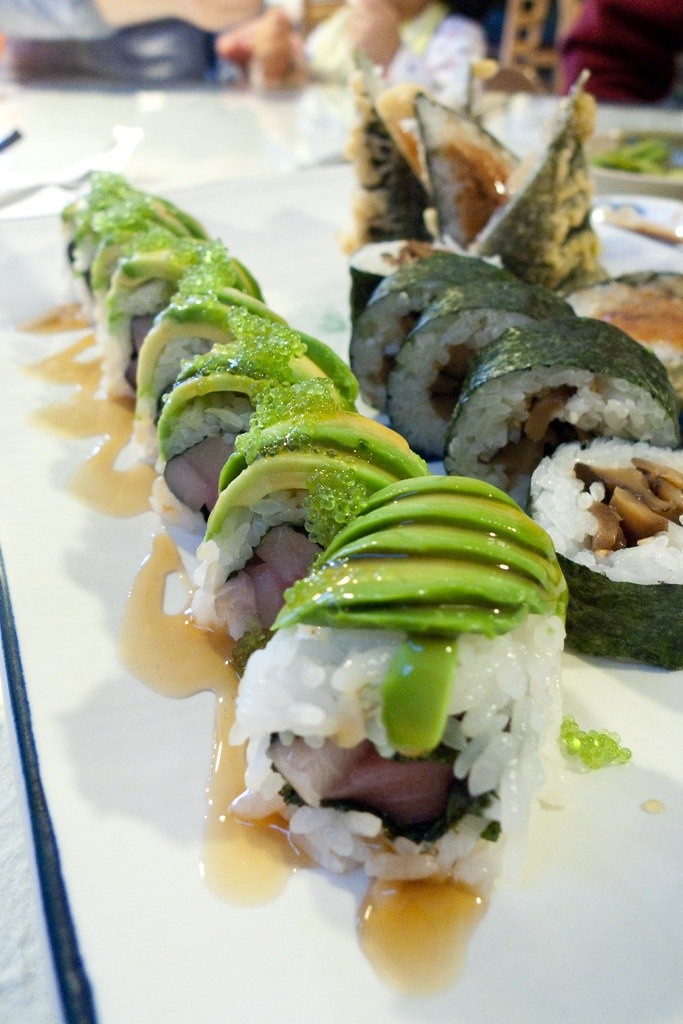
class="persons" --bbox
[216,0,489,111]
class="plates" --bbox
[0,192,683,1024]
[585,129,682,187]
[590,196,683,250]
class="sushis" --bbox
[59,41,683,893]
